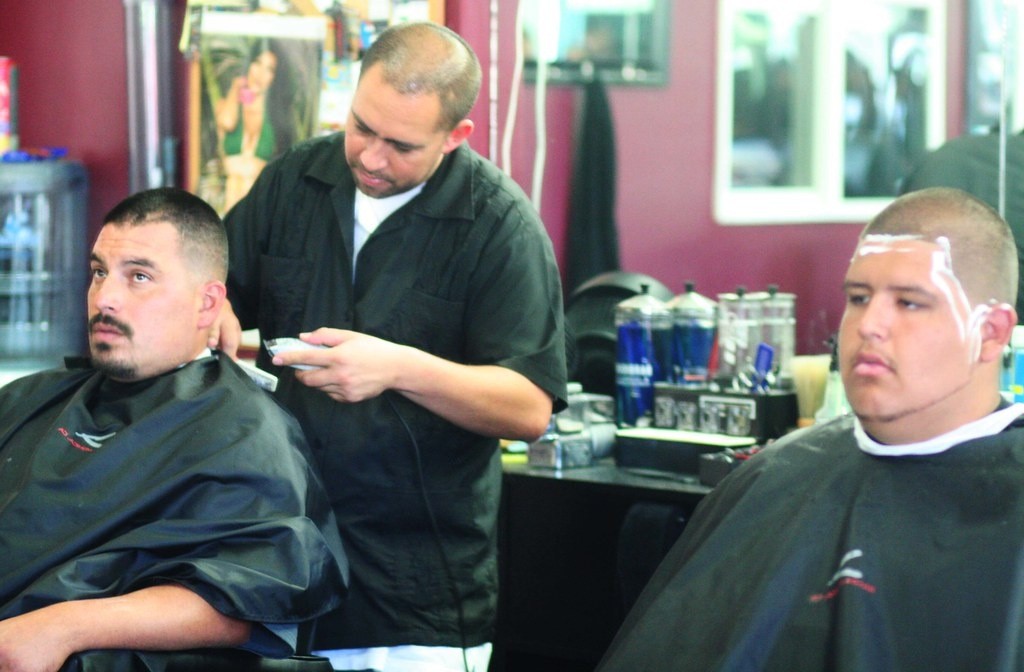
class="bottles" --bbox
[1,161,83,363]
[665,282,715,384]
[614,284,667,432]
[749,285,797,397]
[716,288,762,393]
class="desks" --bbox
[492,459,716,672]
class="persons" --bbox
[205,21,568,672]
[0,187,349,672]
[594,187,1024,672]
